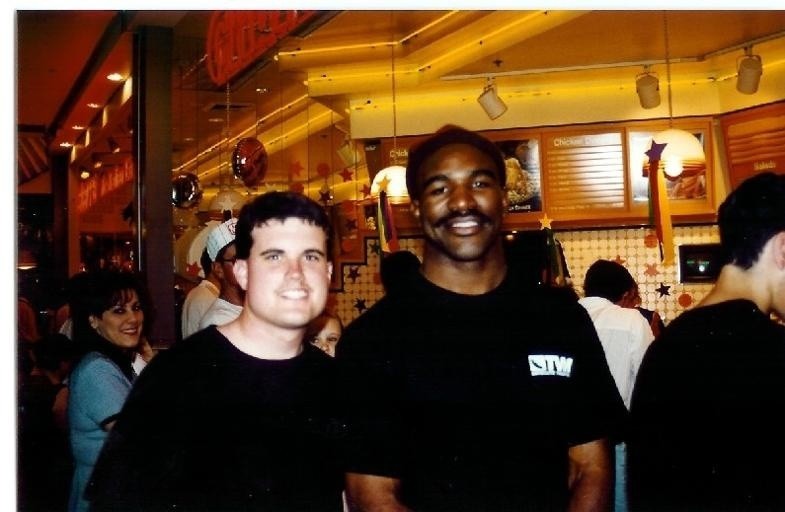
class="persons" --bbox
[79,191,347,512]
[625,171,784,512]
[313,316,345,357]
[502,227,567,289]
[52,275,156,432]
[628,284,668,337]
[65,259,146,511]
[378,251,421,292]
[575,258,656,512]
[201,216,250,331]
[334,124,631,512]
[18,272,72,409]
[178,243,227,338]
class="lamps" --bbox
[18,249,37,271]
[371,10,410,205]
[126,112,134,133]
[208,80,249,212]
[735,44,764,95]
[91,150,133,170]
[636,65,662,109]
[106,134,133,154]
[73,162,91,181]
[644,10,707,179]
[477,75,508,122]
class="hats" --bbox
[205,216,240,265]
